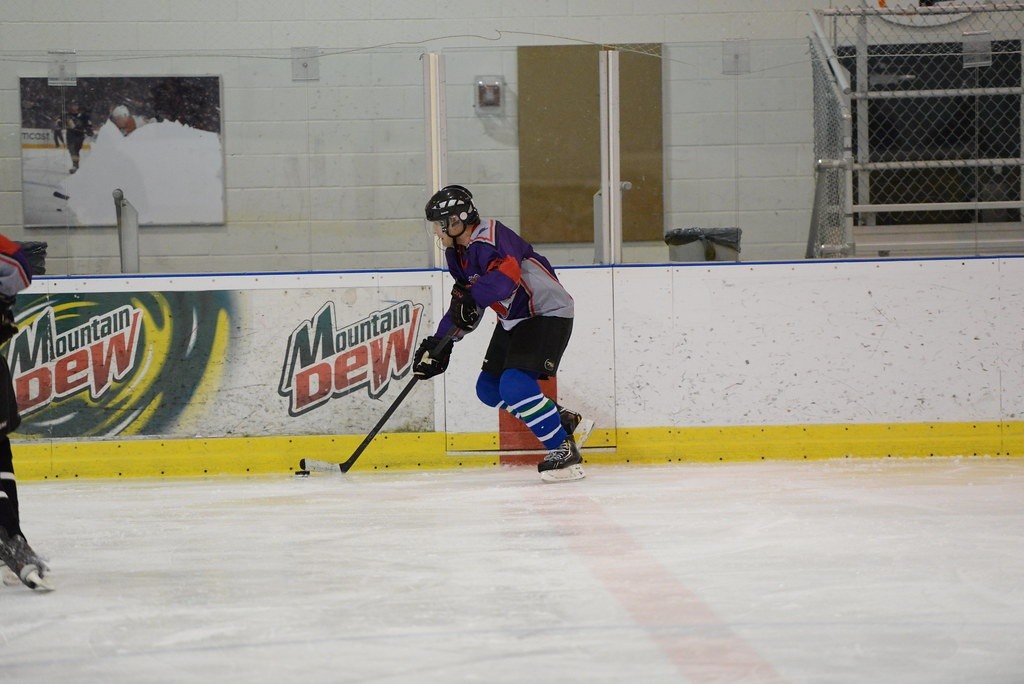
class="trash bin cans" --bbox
[11,241,47,275]
[664,226,743,263]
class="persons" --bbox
[19,77,221,175]
[412,186,595,482]
[0,234,54,593]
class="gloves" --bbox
[449,296,484,333]
[412,334,454,380]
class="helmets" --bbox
[424,184,480,232]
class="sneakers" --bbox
[0,534,54,591]
[559,406,596,450]
[538,435,587,482]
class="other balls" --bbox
[294,471,311,478]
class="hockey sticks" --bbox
[300,324,462,473]
